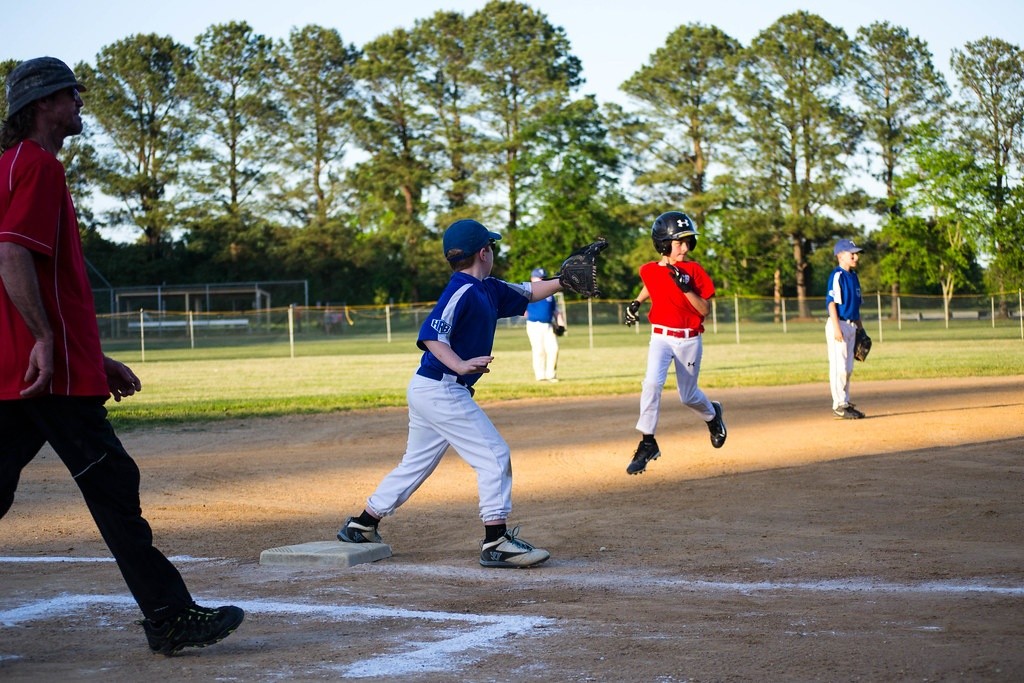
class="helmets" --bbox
[651,212,697,256]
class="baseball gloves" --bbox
[854,328,872,361]
[559,238,609,300]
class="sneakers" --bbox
[626,439,661,475]
[705,401,726,448]
[134,601,244,657]
[337,516,392,552]
[480,527,549,568]
[833,405,865,420]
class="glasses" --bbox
[486,239,496,250]
[46,87,79,102]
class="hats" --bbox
[443,220,502,262]
[531,269,548,278]
[833,240,863,256]
[7,57,86,120]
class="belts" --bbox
[654,327,698,338]
[416,367,474,397]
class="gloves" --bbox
[667,263,692,292]
[624,301,640,327]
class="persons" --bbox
[523,268,565,381]
[625,211,727,475]
[0,57,245,656]
[825,239,870,419]
[336,219,608,567]
